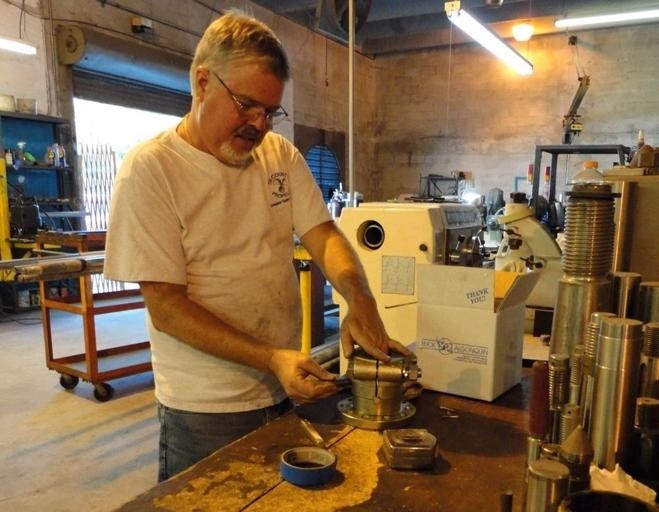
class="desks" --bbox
[109,363,537,512]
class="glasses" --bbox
[215,72,288,125]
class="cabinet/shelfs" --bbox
[35,229,153,400]
[0,110,81,314]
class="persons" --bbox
[100,12,415,484]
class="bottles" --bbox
[525,271,659,512]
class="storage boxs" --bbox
[414,263,544,403]
